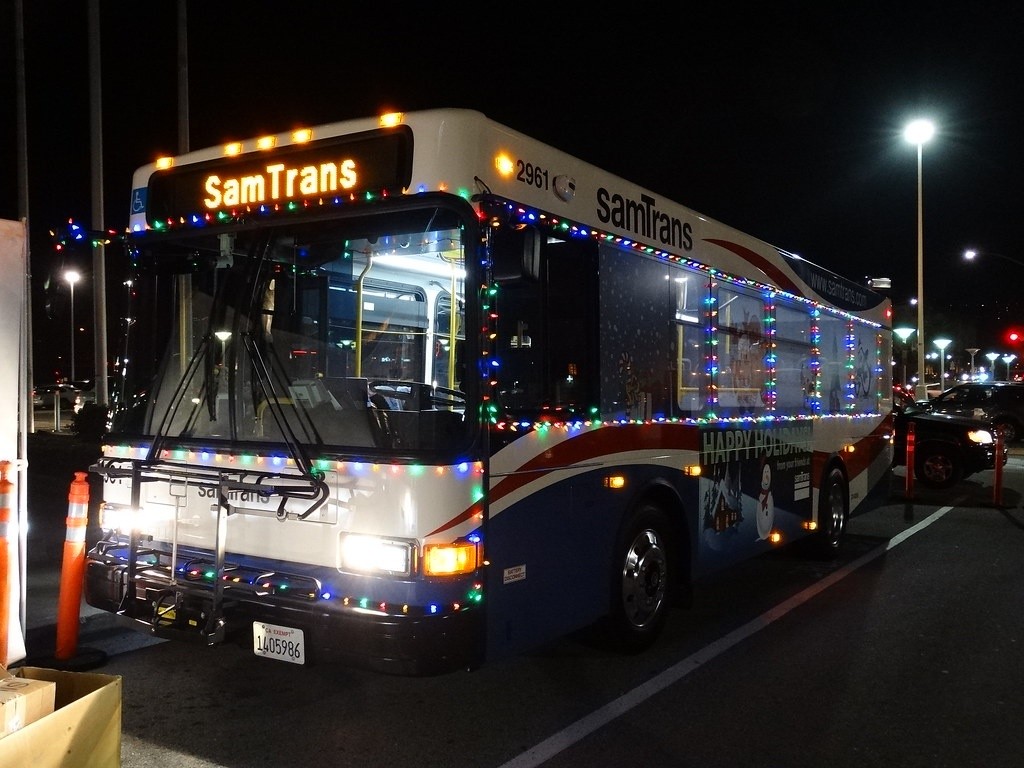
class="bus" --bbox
[79,106,903,693]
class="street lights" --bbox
[56,267,90,380]
[889,326,1019,394]
[886,107,946,408]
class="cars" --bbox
[878,390,1008,499]
[918,380,1024,444]
[32,374,127,418]
